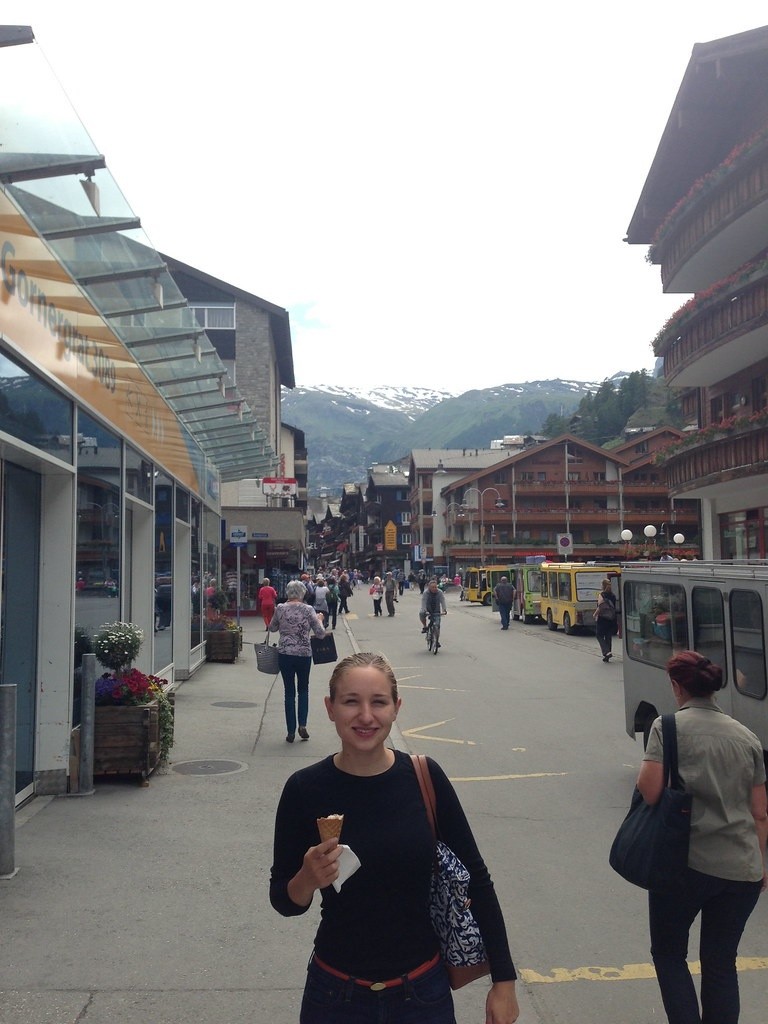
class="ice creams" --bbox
[317,813,344,855]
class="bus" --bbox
[463,564,520,609]
[617,558,768,794]
[538,560,622,636]
[506,563,567,624]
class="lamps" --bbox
[80,169,100,217]
[435,458,447,476]
[251,430,272,488]
[238,403,242,423]
[217,376,225,398]
[152,273,163,309]
[193,336,201,364]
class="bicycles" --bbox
[422,611,446,655]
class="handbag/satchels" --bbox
[254,626,280,674]
[410,754,492,992]
[608,713,693,891]
[311,630,338,664]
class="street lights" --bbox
[458,487,505,568]
[643,525,658,560]
[430,502,467,581]
[674,532,685,559]
[621,528,633,561]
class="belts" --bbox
[312,953,442,990]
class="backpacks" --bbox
[326,585,338,604]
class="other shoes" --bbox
[434,642,442,647]
[421,627,427,633]
[338,611,342,614]
[373,614,378,616]
[387,614,394,617]
[603,654,613,662]
[501,624,508,630]
[332,625,335,629]
[380,610,382,615]
[345,610,350,613]
[323,623,329,628]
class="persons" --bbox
[191,579,219,623]
[419,581,447,634]
[338,574,353,614]
[269,579,325,743]
[301,578,353,630]
[637,648,768,1024]
[259,578,278,631]
[494,576,516,630]
[301,565,465,595]
[370,571,398,617]
[269,652,519,1024]
[659,552,673,561]
[591,578,616,663]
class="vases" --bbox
[93,692,176,787]
[207,630,240,665]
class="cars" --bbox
[151,569,201,613]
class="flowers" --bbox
[75,624,94,667]
[92,621,174,775]
[207,587,238,631]
[652,405,768,469]
[649,255,768,355]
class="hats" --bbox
[501,576,507,582]
[300,573,310,579]
[386,572,393,576]
